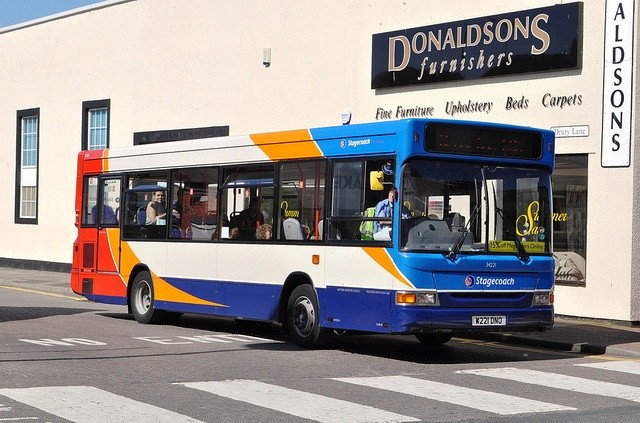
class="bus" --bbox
[70,117,556,344]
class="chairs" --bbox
[283,217,304,240]
[172,225,182,238]
[136,206,146,225]
[202,210,229,225]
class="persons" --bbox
[116,192,139,225]
[165,188,192,223]
[301,225,310,240]
[237,196,264,241]
[373,186,412,241]
[256,224,273,240]
[146,191,166,225]
